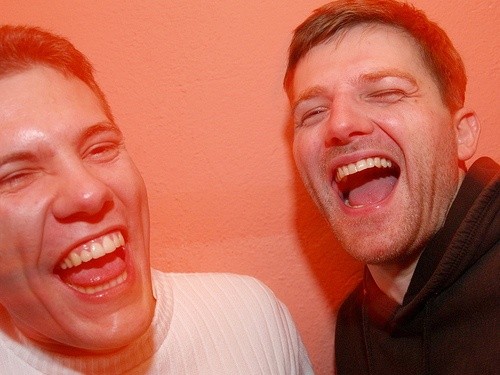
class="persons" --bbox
[283,0,500,375]
[1,21,316,375]
